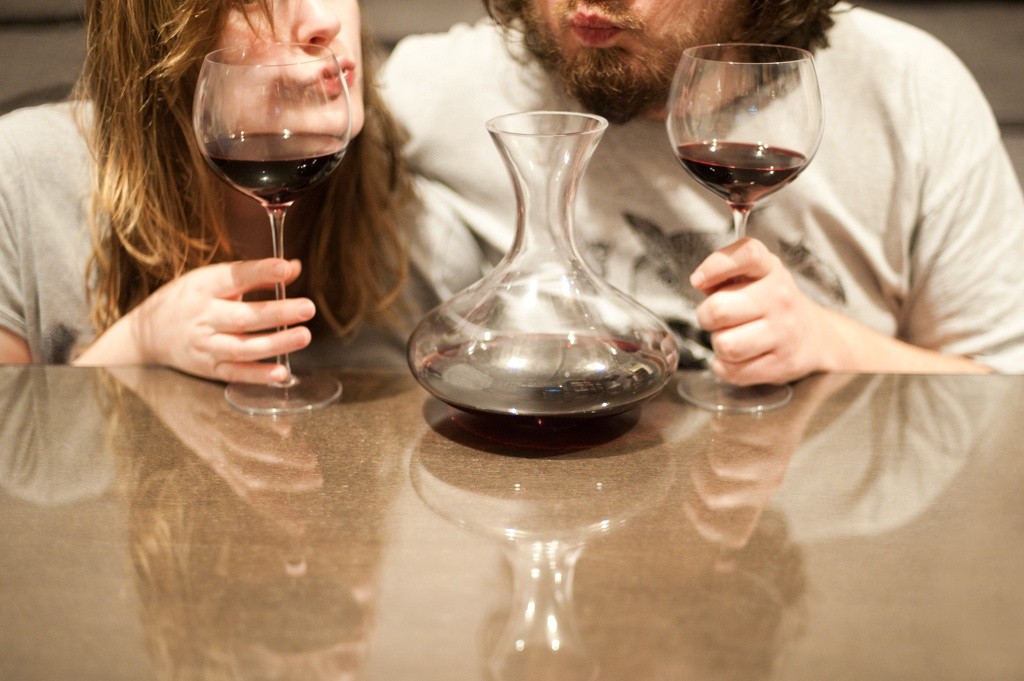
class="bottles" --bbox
[406,111,681,445]
[409,423,681,679]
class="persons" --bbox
[379,0,1024,385]
[0,0,403,384]
[0,367,1024,681]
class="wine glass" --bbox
[193,41,350,416]
[666,43,824,415]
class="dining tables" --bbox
[0,365,1024,681]
